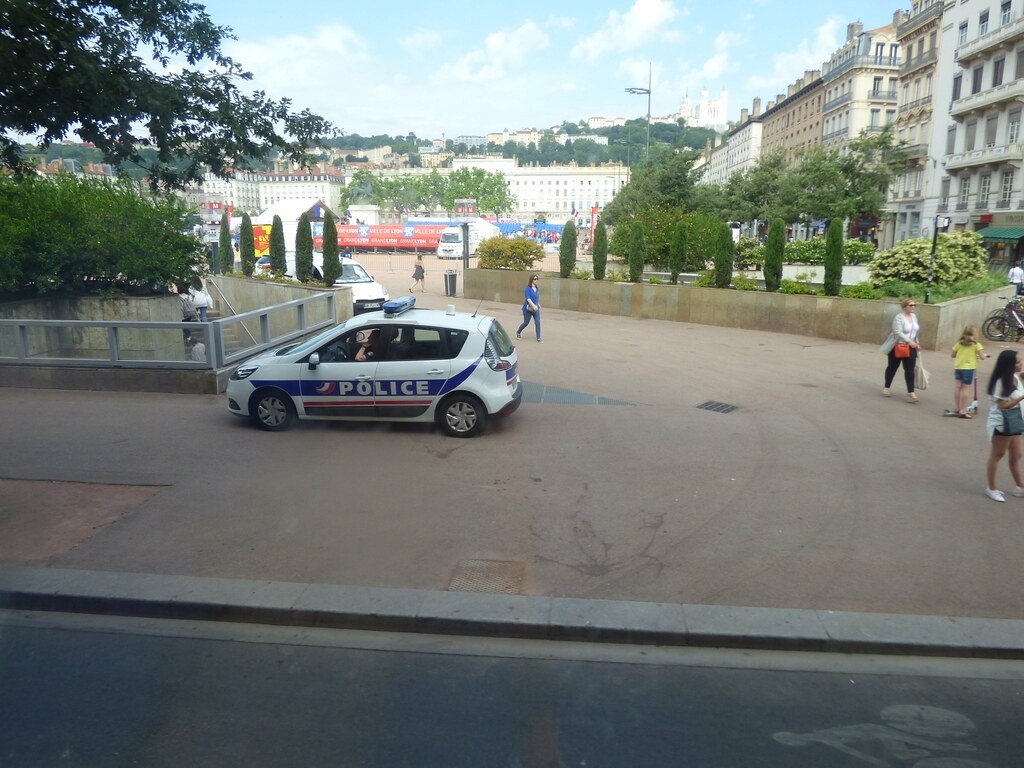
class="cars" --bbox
[226,296,523,439]
[254,250,390,317]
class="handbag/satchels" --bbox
[914,350,928,390]
[527,305,535,312]
[895,343,909,358]
[206,289,213,309]
[1001,406,1024,435]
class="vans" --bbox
[437,227,482,259]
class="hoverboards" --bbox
[944,352,991,419]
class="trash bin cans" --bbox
[444,269,457,295]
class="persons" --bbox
[408,254,427,293]
[1008,261,1024,295]
[508,230,560,245]
[188,276,213,323]
[341,246,352,270]
[516,275,544,344]
[986,350,1024,501]
[582,234,589,248]
[882,297,921,403]
[950,325,988,419]
[355,330,380,361]
[233,242,242,272]
[177,285,199,342]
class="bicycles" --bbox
[981,294,1024,341]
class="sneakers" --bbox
[1013,486,1024,496]
[985,488,1006,501]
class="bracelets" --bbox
[363,345,366,349]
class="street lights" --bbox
[614,61,651,189]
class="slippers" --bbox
[957,408,972,418]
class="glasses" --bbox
[909,304,916,307]
[534,278,538,281]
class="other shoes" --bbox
[907,393,920,403]
[537,338,543,344]
[884,387,891,397]
[422,290,427,293]
[516,332,521,338]
[409,288,412,293]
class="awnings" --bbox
[975,226,1024,243]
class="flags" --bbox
[571,208,578,217]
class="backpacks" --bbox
[180,294,196,319]
[189,284,208,308]
